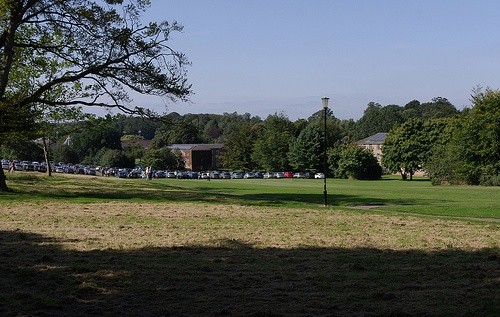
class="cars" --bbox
[1,159,96,175]
[95,165,314,179]
[314,173,324,179]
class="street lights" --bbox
[320,97,331,207]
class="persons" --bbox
[174,170,180,179]
[146,166,154,180]
[8,159,16,174]
